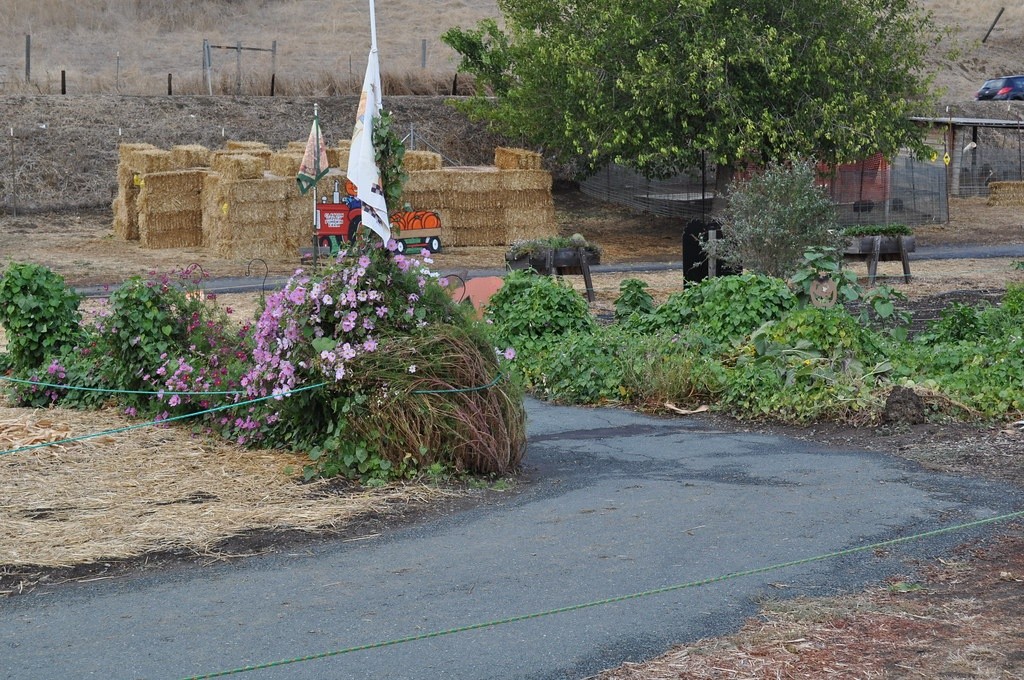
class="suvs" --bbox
[975,75,1024,101]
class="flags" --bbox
[347,48,392,249]
[297,116,330,195]
